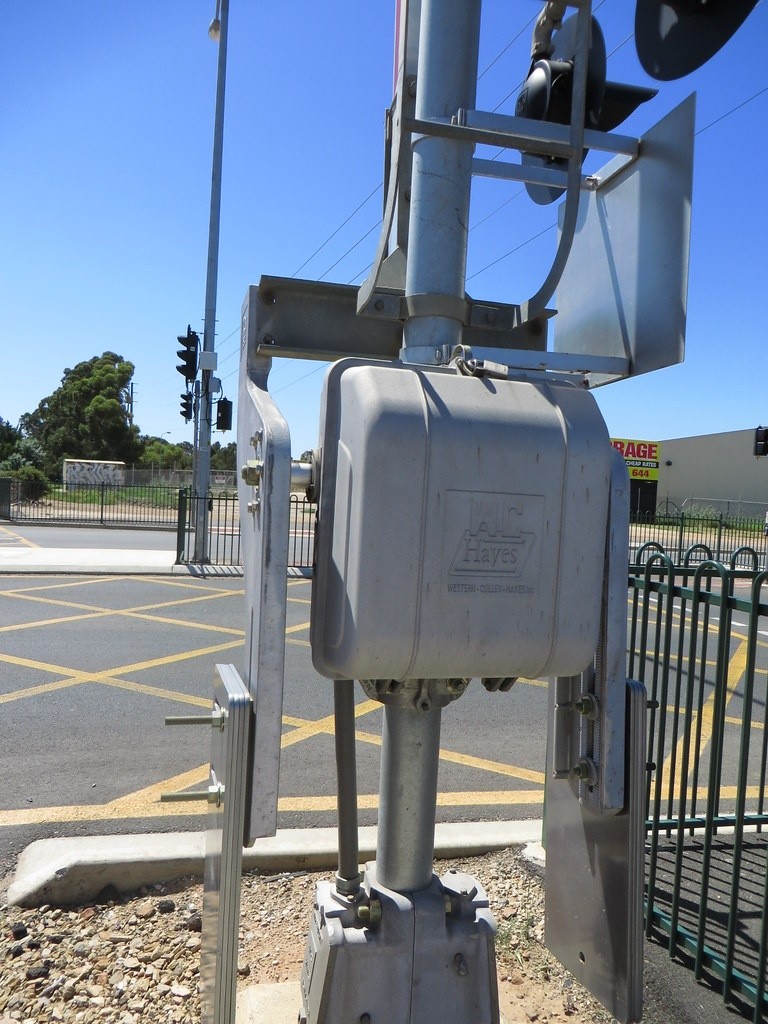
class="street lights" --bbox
[191,1,230,565]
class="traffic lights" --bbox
[179,391,194,422]
[176,325,200,382]
[521,12,659,210]
